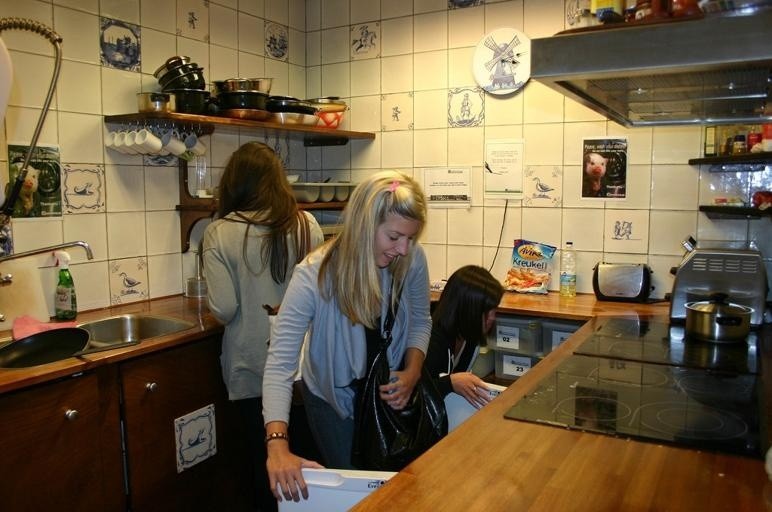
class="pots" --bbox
[1,327,139,368]
[136,92,176,115]
[683,292,756,345]
[151,55,352,129]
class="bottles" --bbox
[596,0,699,25]
[559,243,578,298]
[721,121,762,156]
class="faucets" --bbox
[0,241,94,263]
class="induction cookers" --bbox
[577,317,764,374]
[500,354,771,461]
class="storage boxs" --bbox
[539,319,582,356]
[490,346,537,380]
[487,314,541,357]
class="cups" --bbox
[103,119,206,163]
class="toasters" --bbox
[592,261,655,302]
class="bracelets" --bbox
[264,431,290,441]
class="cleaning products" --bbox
[51,249,77,320]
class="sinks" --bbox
[0,333,102,370]
[76,313,197,348]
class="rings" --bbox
[473,387,476,392]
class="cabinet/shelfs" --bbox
[0,334,226,512]
[101,111,375,257]
[688,150,772,220]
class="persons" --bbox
[202,142,324,512]
[427,266,506,410]
[257,173,431,502]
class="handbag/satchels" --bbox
[353,285,449,472]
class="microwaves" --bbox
[665,233,769,328]
